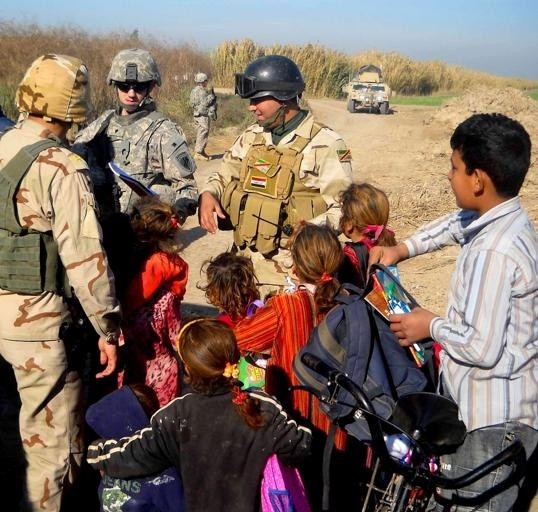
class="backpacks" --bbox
[291,282,427,512]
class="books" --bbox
[108,160,158,198]
[372,267,436,353]
[365,285,434,367]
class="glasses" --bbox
[114,82,150,94]
[232,74,255,96]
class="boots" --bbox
[195,153,212,161]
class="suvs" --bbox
[347,66,389,116]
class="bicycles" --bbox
[284,352,529,510]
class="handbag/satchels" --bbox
[261,452,309,512]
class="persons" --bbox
[190,73,215,160]
[86,316,313,512]
[367,112,538,512]
[72,48,200,226]
[0,53,123,512]
[85,183,399,512]
[197,54,353,299]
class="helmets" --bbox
[194,74,207,83]
[14,53,93,123]
[106,49,161,87]
[244,55,305,101]
